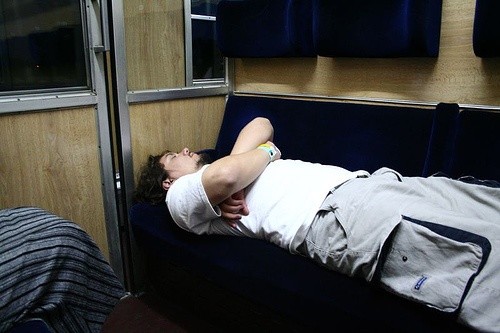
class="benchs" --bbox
[130,90,500,333]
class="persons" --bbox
[0,207,121,333]
[133,117,500,333]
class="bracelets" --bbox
[256,143,276,162]
